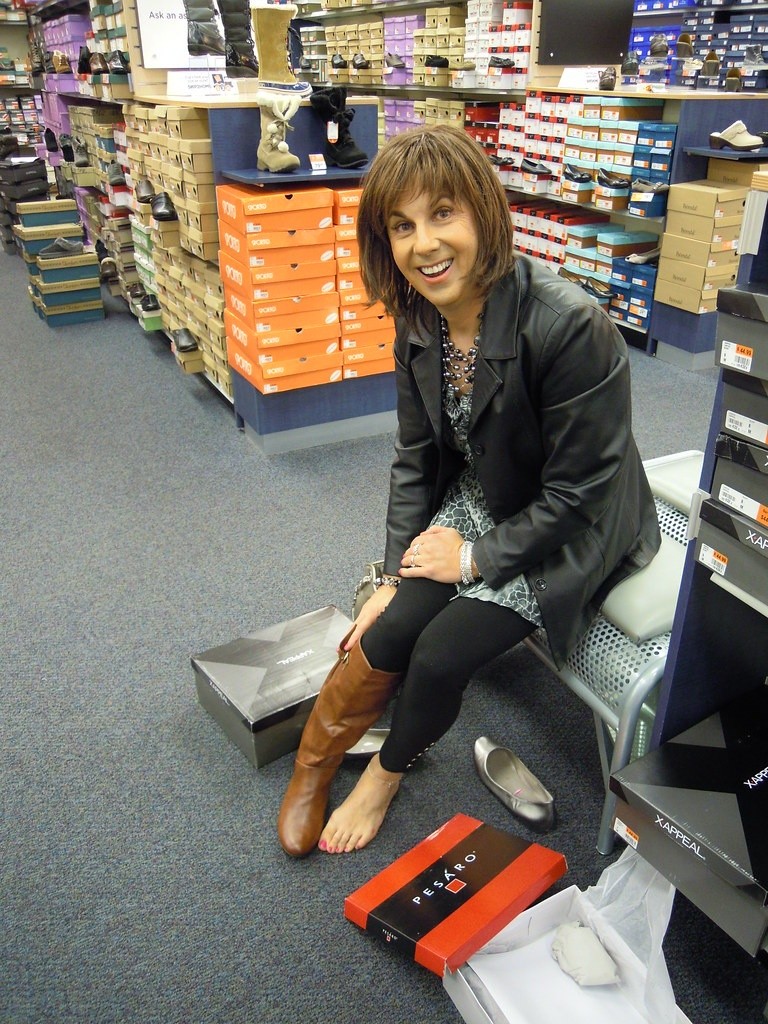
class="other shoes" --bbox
[45,128,58,152]
[298,56,311,69]
[39,237,83,258]
[709,120,764,151]
[72,135,89,167]
[676,33,693,57]
[386,54,405,67]
[96,240,108,262]
[77,46,90,74]
[60,134,74,161]
[700,52,719,75]
[425,55,449,67]
[448,59,475,70]
[725,67,741,92]
[649,33,669,57]
[598,67,616,90]
[331,54,347,68]
[0,136,19,156]
[489,56,515,67]
[622,52,639,74]
[353,54,368,68]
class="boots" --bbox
[312,85,368,169]
[277,635,402,858]
[251,4,311,97]
[254,91,300,172]
[183,0,225,56]
[743,44,767,66]
[218,0,259,77]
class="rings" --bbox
[414,544,420,555]
[410,555,415,566]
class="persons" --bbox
[277,123,661,858]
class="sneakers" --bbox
[107,163,126,186]
[30,46,43,70]
[53,50,72,74]
[42,51,56,73]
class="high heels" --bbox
[625,247,661,265]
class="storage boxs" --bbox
[1,0,768,1024]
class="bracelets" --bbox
[459,539,475,585]
[373,576,399,588]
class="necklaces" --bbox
[438,311,484,407]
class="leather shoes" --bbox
[135,180,156,203]
[474,736,554,832]
[598,169,631,189]
[632,178,670,193]
[100,257,116,277]
[490,154,514,165]
[558,267,584,287]
[520,158,551,174]
[129,282,146,298]
[586,276,612,298]
[141,294,159,311]
[152,192,176,221]
[89,53,109,74]
[565,163,592,183]
[172,328,198,352]
[345,728,391,759]
[109,50,130,74]
